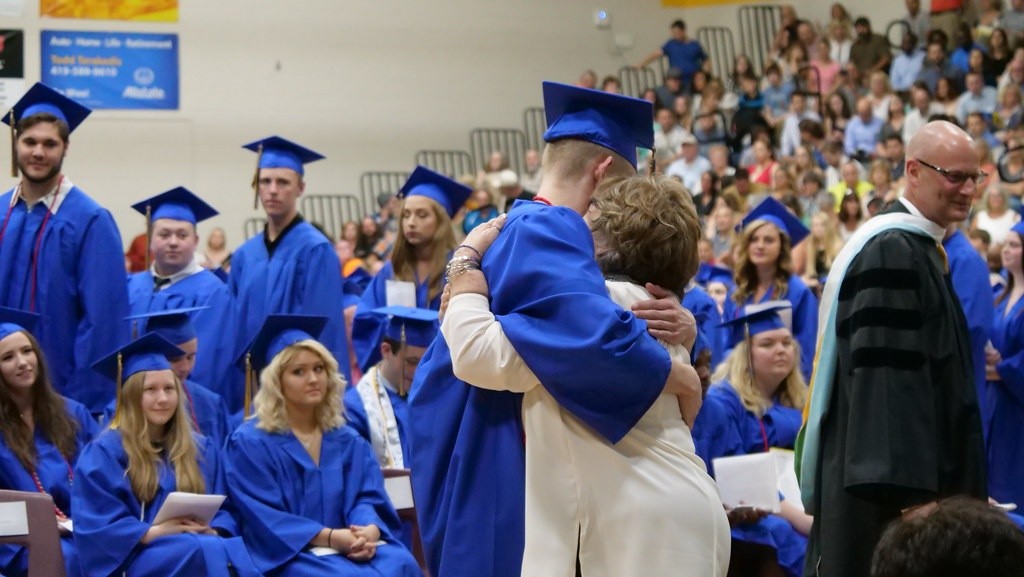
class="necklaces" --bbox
[182,382,199,433]
[531,195,552,206]
[28,458,74,522]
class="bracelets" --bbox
[329,529,333,547]
[456,245,482,258]
[446,256,480,279]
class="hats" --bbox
[396,165,474,219]
[696,264,734,292]
[131,187,220,272]
[233,315,329,418]
[0,306,40,339]
[372,305,439,396]
[733,196,811,247]
[1,83,93,176]
[1012,220,1024,235]
[542,82,657,179]
[89,332,187,431]
[241,135,326,209]
[714,301,793,390]
[123,305,212,347]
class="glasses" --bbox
[916,158,989,186]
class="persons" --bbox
[577,0,1024,577]
[0,77,734,577]
